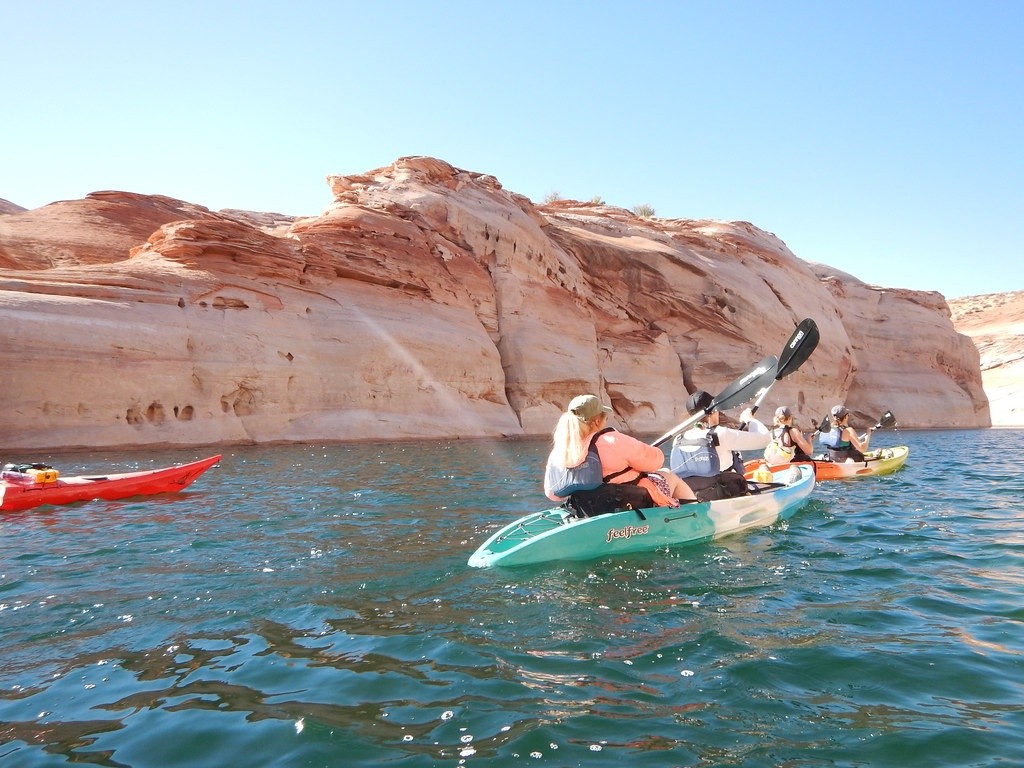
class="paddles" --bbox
[650,355,779,446]
[858,410,896,439]
[812,414,831,437]
[739,318,820,430]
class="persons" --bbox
[669,390,770,503]
[544,395,699,520]
[819,405,875,462]
[769,406,813,461]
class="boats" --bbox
[0,454,224,512]
[466,464,817,568]
[737,445,910,485]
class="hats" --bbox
[775,406,792,416]
[568,394,613,420]
[831,405,850,419]
[685,390,714,411]
